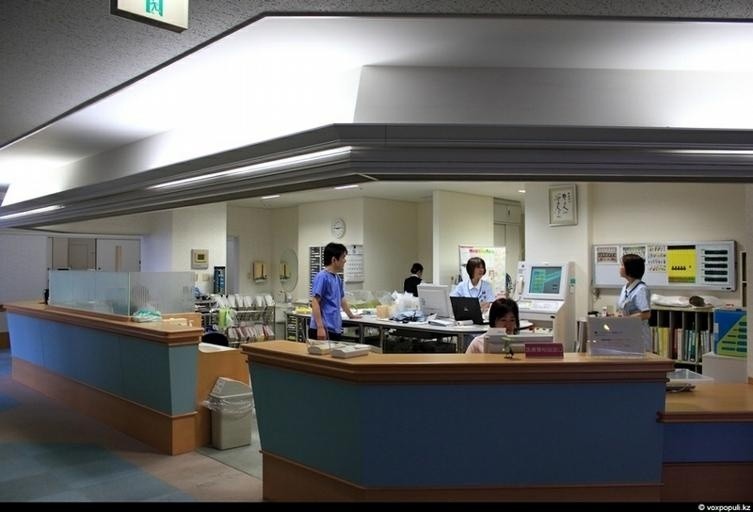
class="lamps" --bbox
[252,260,267,283]
[280,261,291,282]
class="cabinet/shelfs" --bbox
[648,306,715,380]
[194,300,276,348]
[739,251,747,309]
[309,246,344,296]
[591,240,736,291]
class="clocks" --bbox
[331,217,346,237]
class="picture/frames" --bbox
[547,184,578,228]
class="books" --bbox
[650,325,713,363]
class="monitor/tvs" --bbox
[416,283,450,319]
[523,261,568,300]
[483,335,554,353]
[665,370,714,387]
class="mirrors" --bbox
[279,247,298,292]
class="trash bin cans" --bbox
[209,377,253,450]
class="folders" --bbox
[714,310,747,357]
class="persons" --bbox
[309,242,363,341]
[465,298,521,354]
[132,285,156,312]
[615,254,652,352]
[404,261,424,297]
[449,256,496,313]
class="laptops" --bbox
[449,296,489,324]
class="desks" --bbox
[282,308,535,352]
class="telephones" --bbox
[517,277,523,295]
